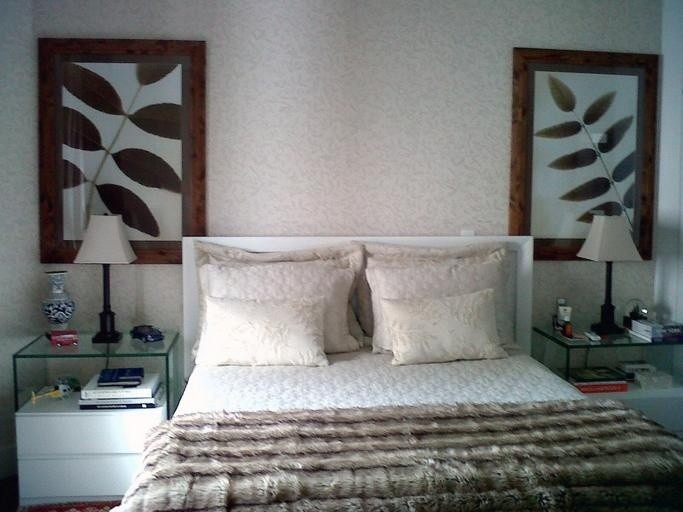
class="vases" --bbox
[39,270,72,339]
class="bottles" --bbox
[563,321,574,339]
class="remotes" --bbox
[584,329,602,341]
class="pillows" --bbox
[193,239,508,370]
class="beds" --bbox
[149,233,681,509]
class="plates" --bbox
[561,331,587,342]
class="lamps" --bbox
[73,212,139,347]
[573,211,647,340]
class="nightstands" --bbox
[532,315,679,446]
[12,333,180,509]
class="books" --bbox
[560,353,674,394]
[77,367,165,409]
[624,316,682,344]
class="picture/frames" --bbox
[35,35,208,266]
[508,46,660,264]
